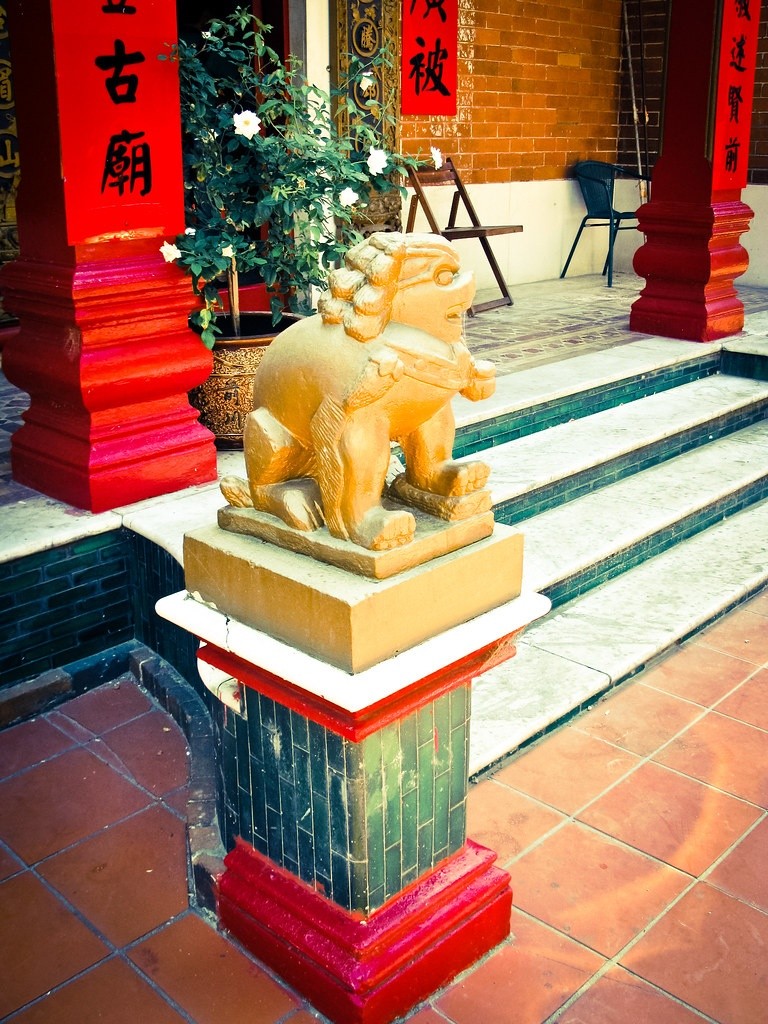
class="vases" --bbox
[188,310,307,450]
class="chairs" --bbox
[561,160,652,287]
[406,157,523,318]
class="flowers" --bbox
[158,6,444,349]
